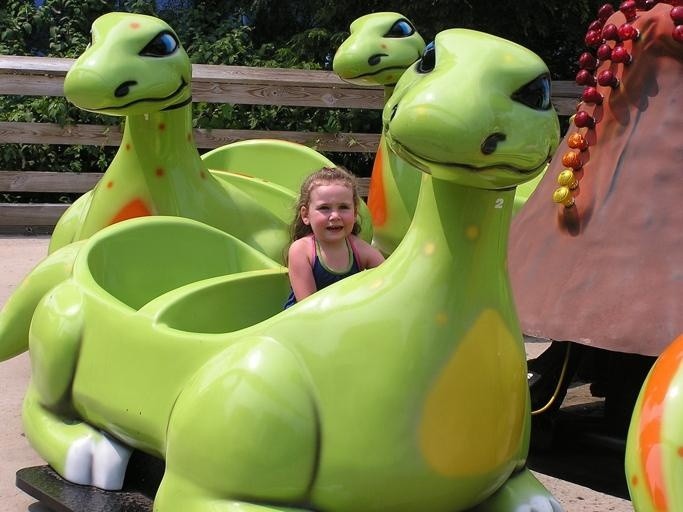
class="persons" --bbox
[282,167,385,311]
